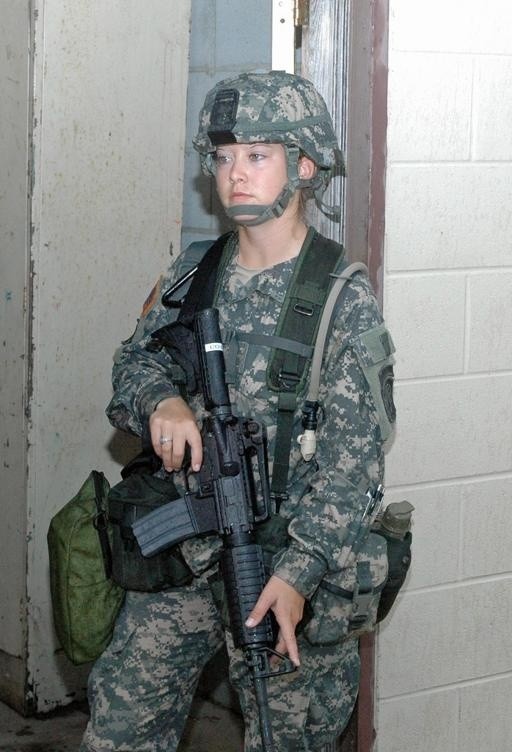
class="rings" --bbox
[160,435,174,446]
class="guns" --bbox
[131,310,276,752]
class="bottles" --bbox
[372,501,415,623]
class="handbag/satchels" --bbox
[48,470,191,666]
[302,528,412,648]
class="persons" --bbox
[75,67,400,748]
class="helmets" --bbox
[193,72,344,178]
